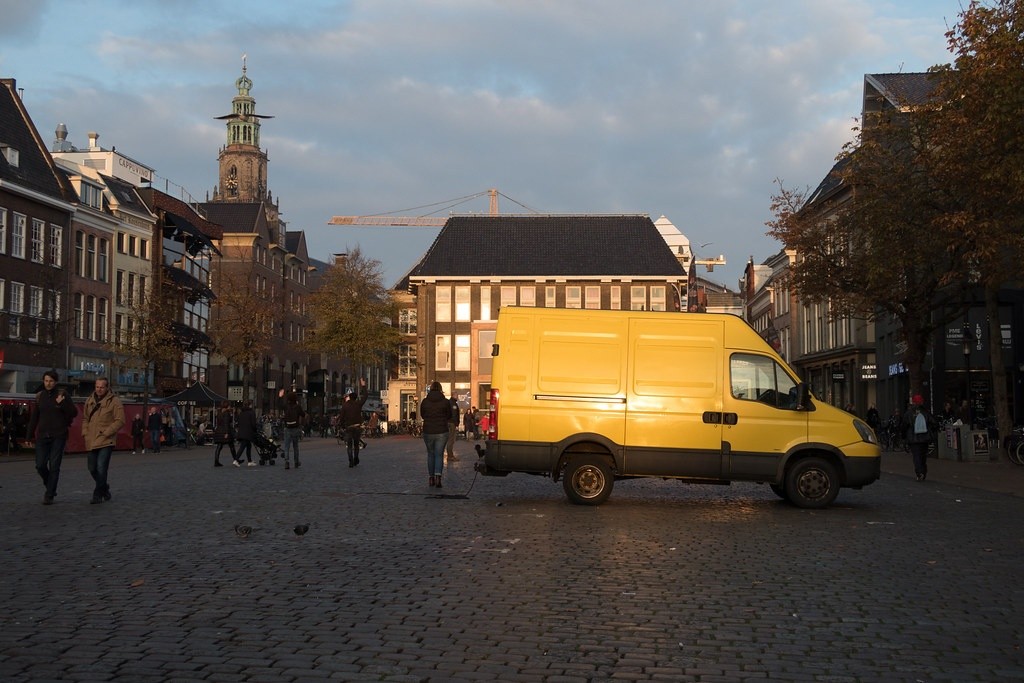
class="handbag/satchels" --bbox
[160,433,165,442]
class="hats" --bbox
[912,395,924,405]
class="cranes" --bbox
[329,188,498,227]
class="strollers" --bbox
[253,431,288,466]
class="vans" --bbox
[474,306,888,508]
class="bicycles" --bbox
[334,420,423,449]
[879,429,936,455]
[1008,426,1024,465]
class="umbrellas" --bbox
[162,380,228,417]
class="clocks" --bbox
[225,175,238,189]
[258,177,267,193]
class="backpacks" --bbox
[911,408,928,434]
[284,404,299,428]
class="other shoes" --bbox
[90,497,102,504]
[349,461,354,467]
[295,462,301,468]
[232,460,241,467]
[132,451,136,455]
[917,472,926,481]
[152,451,157,454]
[284,462,289,469]
[141,448,145,454]
[354,458,360,466]
[43,495,54,504]
[248,460,258,467]
[239,459,245,464]
[214,462,223,467]
[429,477,435,486]
[447,457,460,462]
[157,450,160,455]
[102,483,111,501]
[435,475,442,487]
[363,443,367,448]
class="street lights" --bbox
[962,342,977,413]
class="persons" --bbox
[23,370,79,506]
[212,400,245,467]
[361,411,380,439]
[130,413,145,454]
[340,378,369,468]
[936,398,977,431]
[81,377,125,504]
[146,406,164,454]
[420,382,452,486]
[198,419,210,434]
[233,398,257,467]
[901,394,940,481]
[280,392,306,468]
[447,397,460,461]
[843,401,858,417]
[865,403,881,429]
[463,406,489,441]
[302,411,342,438]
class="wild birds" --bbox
[293,523,311,537]
[234,524,253,538]
